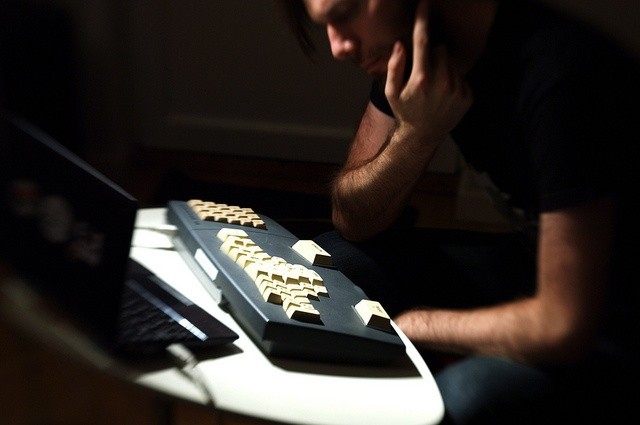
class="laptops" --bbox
[0,118,239,361]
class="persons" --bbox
[306,0,616,425]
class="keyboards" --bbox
[168,200,406,370]
